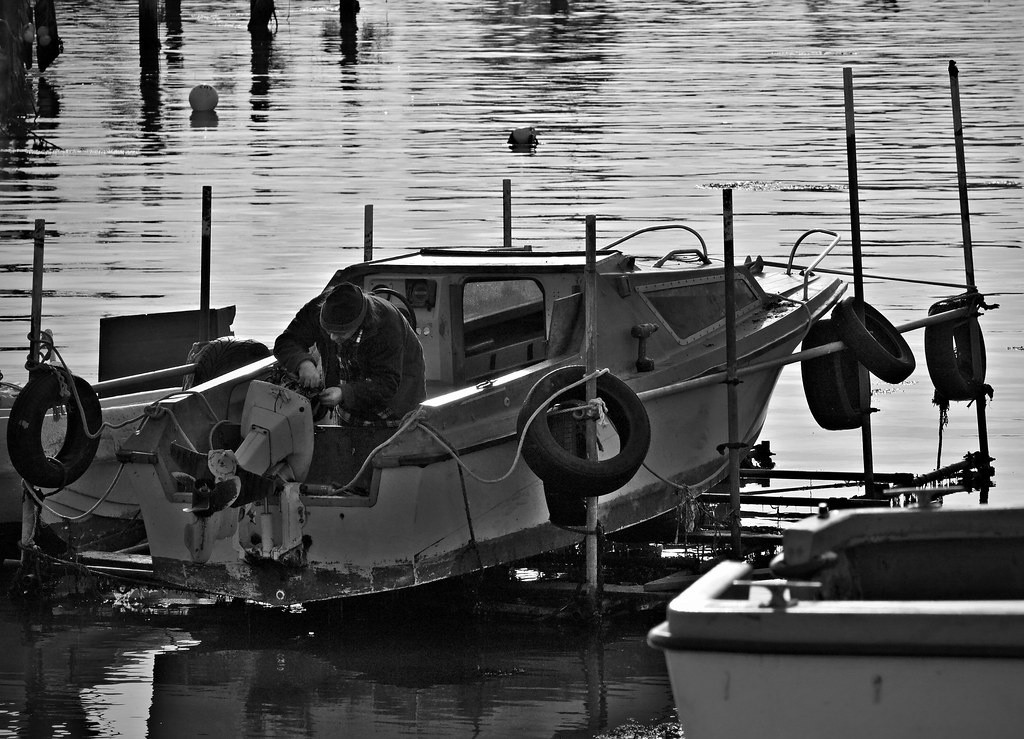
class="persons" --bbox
[272,279,428,493]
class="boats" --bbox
[1,242,1024,739]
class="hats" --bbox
[319,282,368,333]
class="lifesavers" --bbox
[516,364,653,498]
[832,295,916,385]
[924,304,987,401]
[193,335,270,388]
[7,370,102,489]
[800,320,872,430]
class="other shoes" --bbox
[342,481,369,497]
